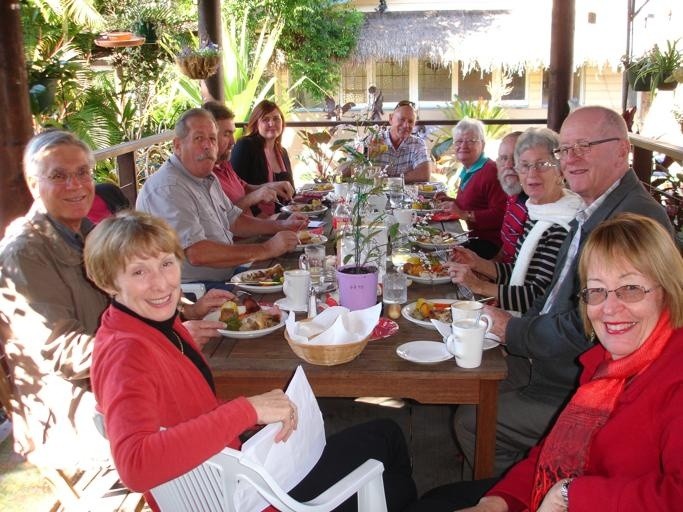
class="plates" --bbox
[396,180,467,285]
[230,270,282,296]
[446,331,499,349]
[274,297,310,314]
[203,304,288,339]
[94,38,147,48]
[279,182,350,304]
[399,297,462,329]
[397,340,454,365]
[369,317,398,342]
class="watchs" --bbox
[560,477,571,508]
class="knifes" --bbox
[223,279,280,287]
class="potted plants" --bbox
[621,53,651,93]
[333,183,394,309]
[625,42,681,93]
[27,60,61,115]
[177,34,222,81]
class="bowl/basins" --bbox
[108,32,134,40]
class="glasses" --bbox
[516,160,557,174]
[583,284,663,306]
[553,138,621,160]
[394,100,416,110]
[453,138,477,146]
[49,168,94,182]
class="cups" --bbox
[381,273,408,304]
[446,320,484,369]
[450,301,493,335]
[387,171,417,265]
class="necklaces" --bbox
[172,331,183,356]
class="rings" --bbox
[289,414,294,422]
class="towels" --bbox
[231,361,329,511]
[279,302,384,349]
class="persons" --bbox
[82,212,415,512]
[441,213,682,512]
[433,116,508,259]
[452,107,678,478]
[137,100,308,293]
[1,132,151,512]
[337,103,430,183]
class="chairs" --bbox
[99,412,387,512]
[89,183,134,225]
[0,356,145,512]
